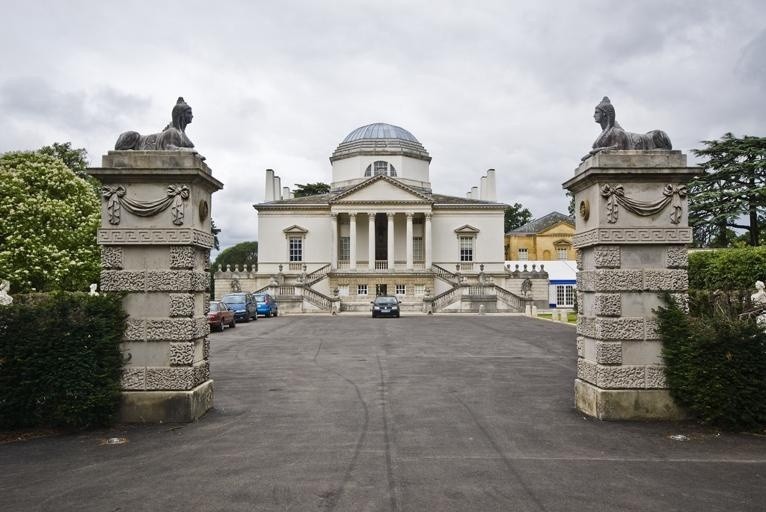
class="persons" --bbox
[581,96,672,161]
[0,280,13,305]
[751,281,766,303]
[115,97,206,160]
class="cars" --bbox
[370,295,402,318]
[207,293,278,331]
